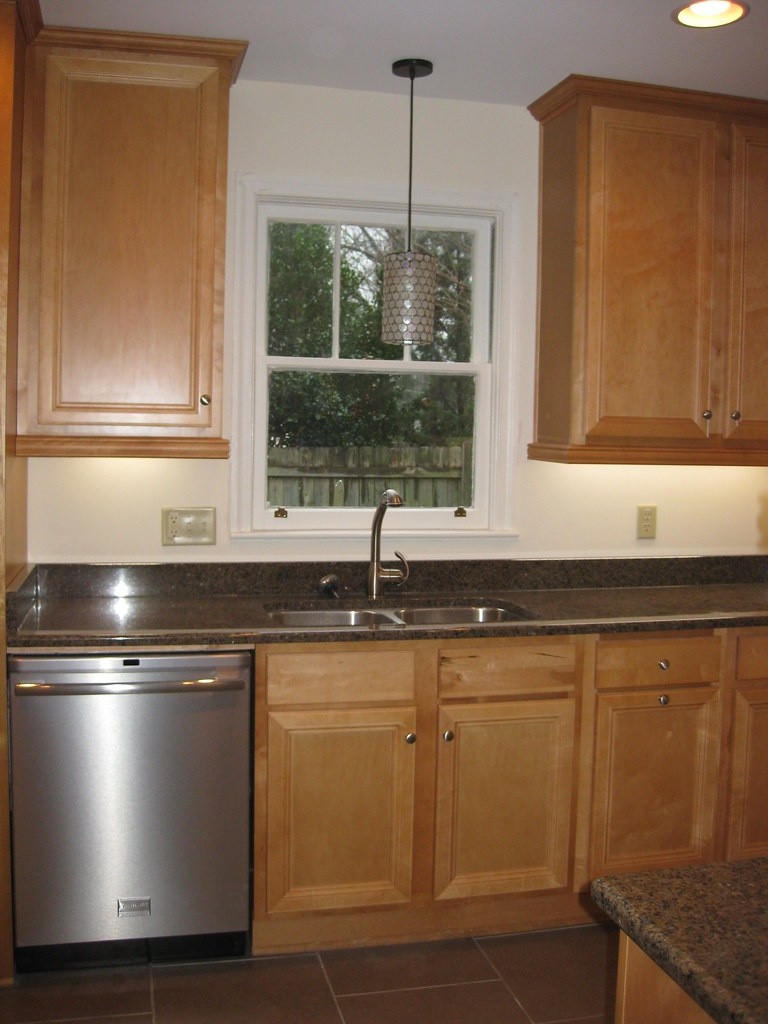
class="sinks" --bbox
[269,610,396,631]
[395,608,526,625]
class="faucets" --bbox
[369,489,407,597]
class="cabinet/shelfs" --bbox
[253,642,584,958]
[587,626,725,927]
[526,73,768,468]
[722,626,768,862]
[13,22,250,460]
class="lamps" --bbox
[670,0,752,30]
[379,58,437,346]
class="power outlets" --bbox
[161,506,217,545]
[636,505,657,539]
